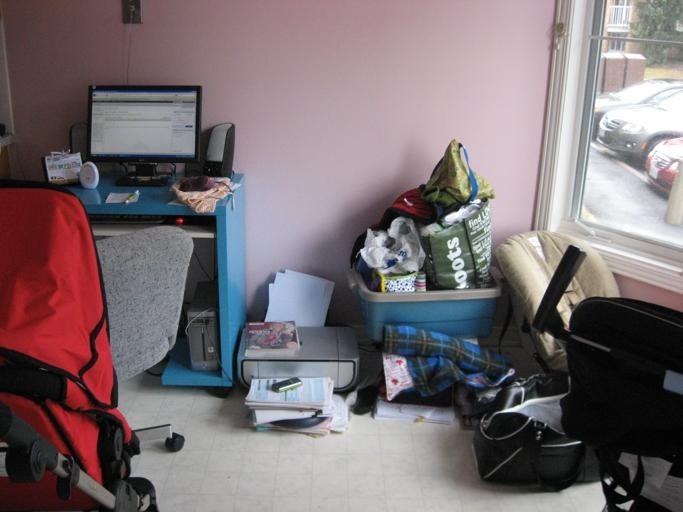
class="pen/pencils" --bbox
[125,190,140,204]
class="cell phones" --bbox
[272,377,303,391]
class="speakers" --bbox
[69,123,89,158]
[204,122,236,178]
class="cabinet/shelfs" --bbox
[62,170,247,398]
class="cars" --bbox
[591,79,683,196]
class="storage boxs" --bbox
[346,266,504,343]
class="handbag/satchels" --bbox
[473,379,623,491]
[418,138,494,206]
[558,296,682,455]
[418,197,493,289]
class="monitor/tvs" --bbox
[85,82,204,186]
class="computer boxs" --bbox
[235,326,360,394]
[187,280,220,372]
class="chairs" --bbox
[94,225,195,452]
[496,229,622,373]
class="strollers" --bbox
[0,178,156,512]
[531,244,682,511]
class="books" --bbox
[243,321,347,436]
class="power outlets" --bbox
[121,0,141,25]
